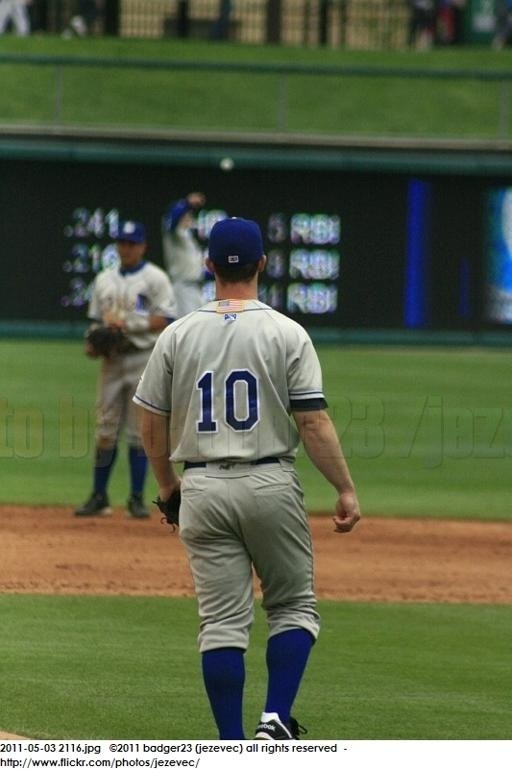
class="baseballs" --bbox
[220,158,234,169]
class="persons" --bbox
[401,1,447,49]
[160,192,215,319]
[72,215,176,521]
[0,1,35,36]
[491,1,512,50]
[126,213,365,740]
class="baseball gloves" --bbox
[85,323,154,355]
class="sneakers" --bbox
[254,712,307,740]
[128,495,150,518]
[75,492,109,515]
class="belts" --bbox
[183,457,280,470]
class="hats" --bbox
[115,223,144,241]
[208,216,262,263]
